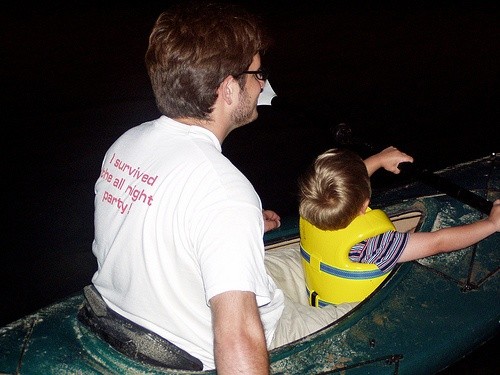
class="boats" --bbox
[0,149,500,375]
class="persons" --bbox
[298,147,500,308]
[92,6,362,375]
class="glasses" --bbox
[233,67,269,80]
